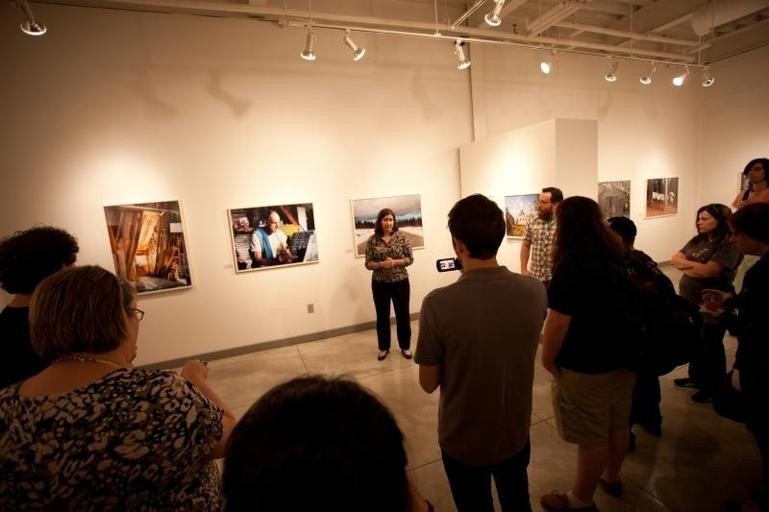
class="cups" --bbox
[128,307,144,320]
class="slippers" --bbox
[626,261,675,341]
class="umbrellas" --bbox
[401,349,412,358]
[377,349,388,360]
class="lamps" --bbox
[19,3,47,36]
[671,68,691,88]
[485,1,505,26]
[343,35,366,61]
[301,31,317,61]
[704,67,713,86]
[455,42,471,70]
[605,62,618,82]
[540,54,560,74]
[640,65,657,85]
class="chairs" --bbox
[540,492,598,511]
[674,378,699,388]
[597,475,623,496]
[691,390,712,402]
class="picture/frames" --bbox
[227,203,319,273]
[504,194,539,239]
[644,177,678,218]
[350,194,423,257]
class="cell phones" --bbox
[436,257,461,273]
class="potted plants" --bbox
[504,194,539,239]
[644,177,678,218]
[350,194,423,257]
[227,203,319,273]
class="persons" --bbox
[1,265,237,512]
[520,188,563,342]
[365,209,414,360]
[0,226,79,392]
[733,159,767,208]
[606,216,663,452]
[673,203,739,403]
[701,201,766,511]
[540,195,644,512]
[249,210,289,262]
[220,373,412,512]
[412,194,549,512]
